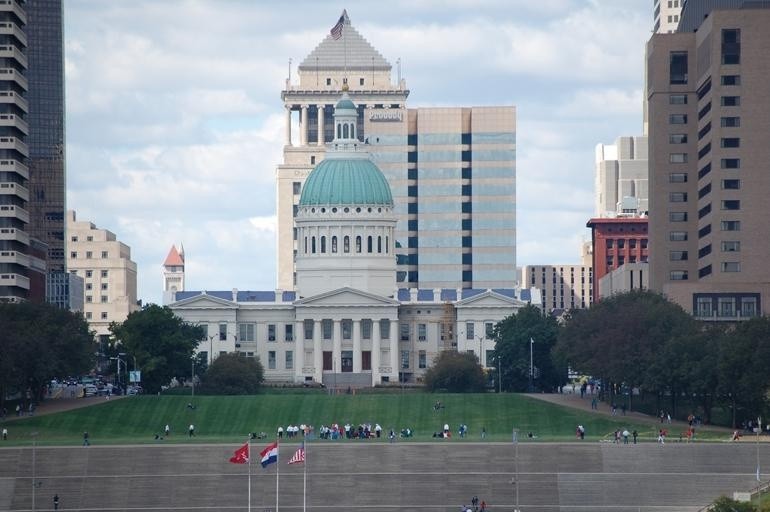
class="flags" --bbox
[228,441,249,464]
[259,440,277,469]
[287,437,304,465]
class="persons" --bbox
[154,422,170,440]
[569,377,770,445]
[437,400,440,410]
[513,423,519,442]
[433,403,438,416]
[54,493,60,510]
[189,424,196,438]
[460,494,487,512]
[277,424,415,439]
[1,384,55,439]
[83,432,91,445]
[481,423,486,438]
[526,431,538,439]
[431,421,469,438]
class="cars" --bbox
[127,385,144,395]
[50,374,115,397]
[303,382,326,389]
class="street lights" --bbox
[208,333,218,366]
[118,351,136,386]
[228,332,238,352]
[753,427,763,511]
[512,427,522,512]
[109,356,128,385]
[30,431,37,511]
[497,356,502,393]
[474,332,485,368]
[449,329,464,352]
